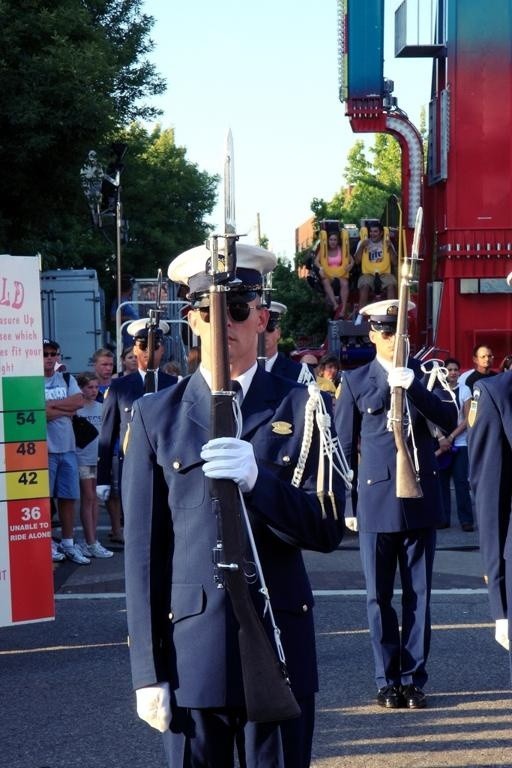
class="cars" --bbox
[290,341,329,362]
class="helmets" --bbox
[300,355,318,365]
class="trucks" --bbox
[38,266,110,379]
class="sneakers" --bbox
[52,540,115,565]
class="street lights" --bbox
[80,148,125,378]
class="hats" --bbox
[264,296,288,321]
[358,297,415,327]
[55,360,66,372]
[44,337,60,349]
[166,238,278,299]
[126,317,169,341]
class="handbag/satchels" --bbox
[62,370,99,450]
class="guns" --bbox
[209,129,303,722]
[390,206,425,500]
[145,269,165,394]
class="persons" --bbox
[468,369,512,651]
[42,285,186,564]
[262,301,343,403]
[121,240,355,766]
[314,222,398,326]
[331,299,458,707]
[443,346,511,532]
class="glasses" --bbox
[308,364,318,368]
[371,329,394,340]
[43,351,57,358]
[480,354,495,359]
[267,318,276,332]
[139,342,159,351]
[197,303,256,322]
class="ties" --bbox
[229,379,242,391]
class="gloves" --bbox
[387,366,416,389]
[96,484,113,502]
[343,516,359,533]
[200,436,258,494]
[134,682,177,735]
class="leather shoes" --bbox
[400,684,426,709]
[463,523,473,531]
[377,684,405,708]
